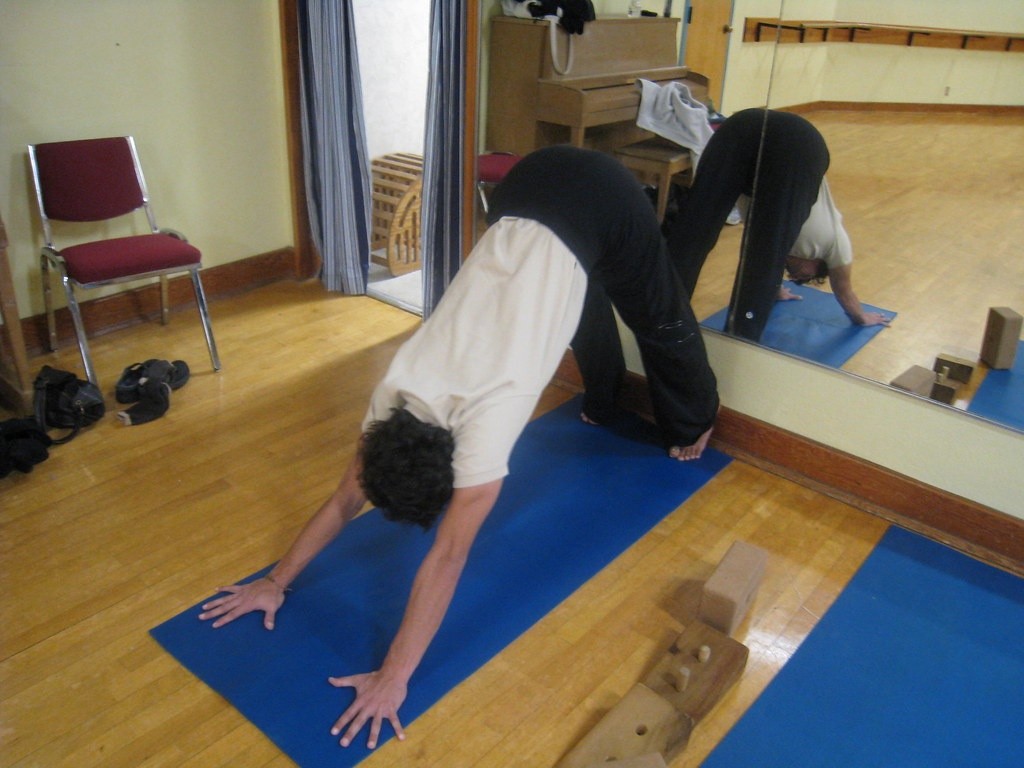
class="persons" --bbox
[198,146,722,749]
[668,106,892,344]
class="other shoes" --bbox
[112,359,190,404]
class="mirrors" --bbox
[460,0,1024,560]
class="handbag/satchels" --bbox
[32,364,106,445]
[500,0,597,76]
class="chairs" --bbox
[27,136,221,390]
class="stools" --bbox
[611,136,693,224]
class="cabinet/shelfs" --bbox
[485,14,709,154]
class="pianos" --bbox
[483,16,724,226]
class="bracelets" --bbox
[264,574,292,592]
